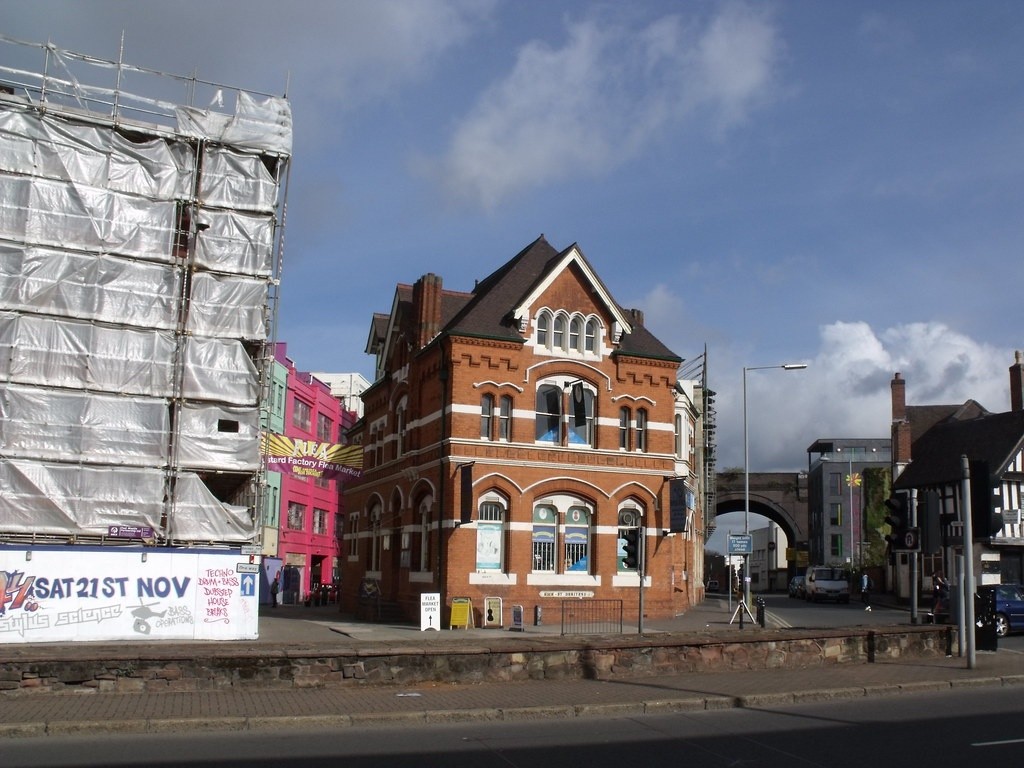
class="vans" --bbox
[807,566,849,602]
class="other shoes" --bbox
[929,614,934,616]
[869,609,871,611]
[865,606,870,610]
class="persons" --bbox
[931,571,951,625]
[861,568,873,612]
[271,578,279,608]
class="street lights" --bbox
[897,488,919,623]
[743,364,808,609]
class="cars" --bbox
[788,576,806,598]
[935,582,1024,638]
[706,581,720,592]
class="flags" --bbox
[546,390,559,432]
[572,382,587,428]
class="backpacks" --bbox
[867,577,873,591]
